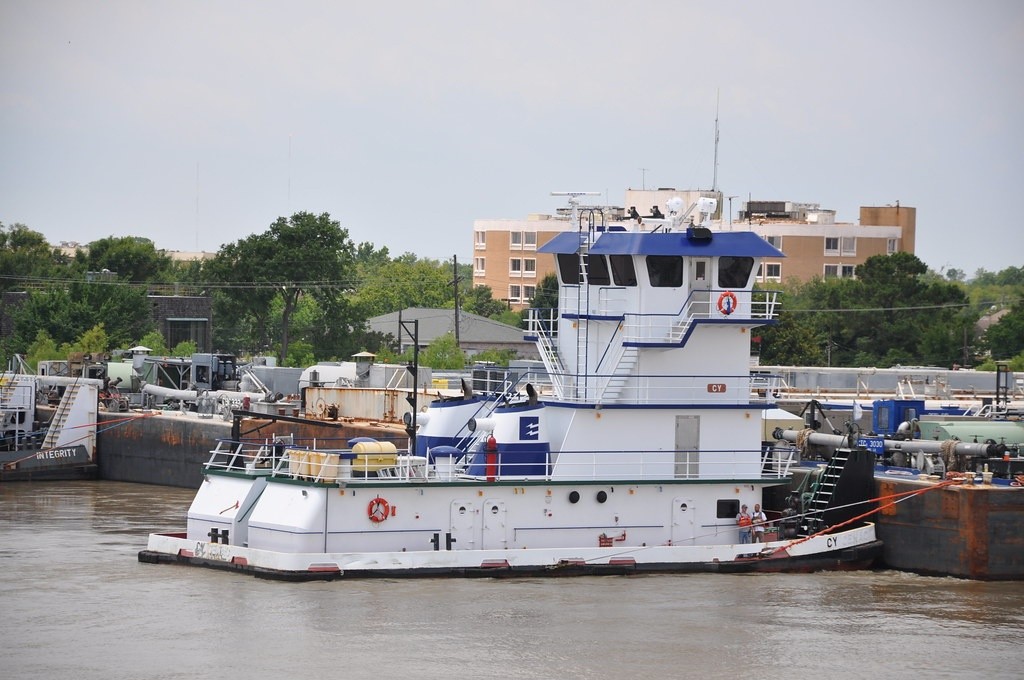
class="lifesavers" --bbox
[367,499,389,523]
[718,291,737,315]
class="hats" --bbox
[742,504,748,508]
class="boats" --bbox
[138,196,886,583]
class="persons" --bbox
[751,504,767,543]
[736,504,752,544]
